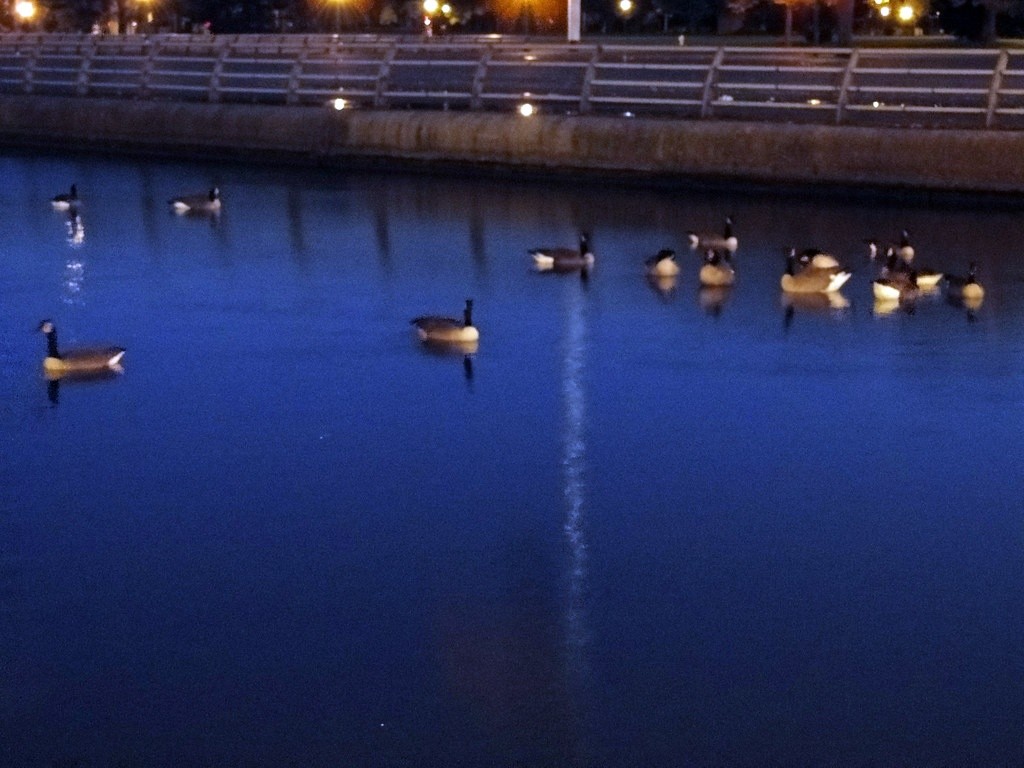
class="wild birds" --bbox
[407,297,480,344]
[32,318,127,381]
[642,214,986,304]
[416,345,480,393]
[49,181,83,210]
[167,185,222,210]
[526,229,597,262]
